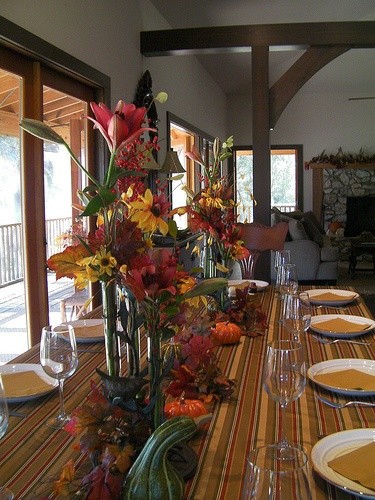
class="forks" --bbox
[313,336,371,345]
[318,397,375,409]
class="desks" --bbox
[348,232,375,280]
[0,284,375,500]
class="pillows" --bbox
[278,213,308,240]
[301,216,324,239]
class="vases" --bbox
[146,328,162,384]
[202,241,218,280]
[102,281,121,381]
[123,287,141,380]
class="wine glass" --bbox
[280,291,311,370]
[0,373,14,500]
[263,340,307,460]
[40,325,78,429]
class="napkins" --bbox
[327,442,375,492]
[310,317,372,334]
[0,369,56,399]
[229,280,253,291]
[61,325,104,340]
[314,369,375,392]
[308,291,357,302]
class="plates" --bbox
[0,364,59,403]
[310,315,375,337]
[307,359,375,395]
[311,428,375,499]
[53,319,124,343]
[227,280,269,291]
[300,289,359,305]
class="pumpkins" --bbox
[120,413,212,500]
[164,398,208,432]
[328,222,342,233]
[210,321,241,344]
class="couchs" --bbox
[271,211,339,286]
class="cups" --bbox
[274,250,298,295]
[240,445,313,500]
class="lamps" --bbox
[163,149,187,180]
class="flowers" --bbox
[18,87,266,500]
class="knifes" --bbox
[52,347,102,353]
[9,412,26,416]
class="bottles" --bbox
[249,283,257,296]
